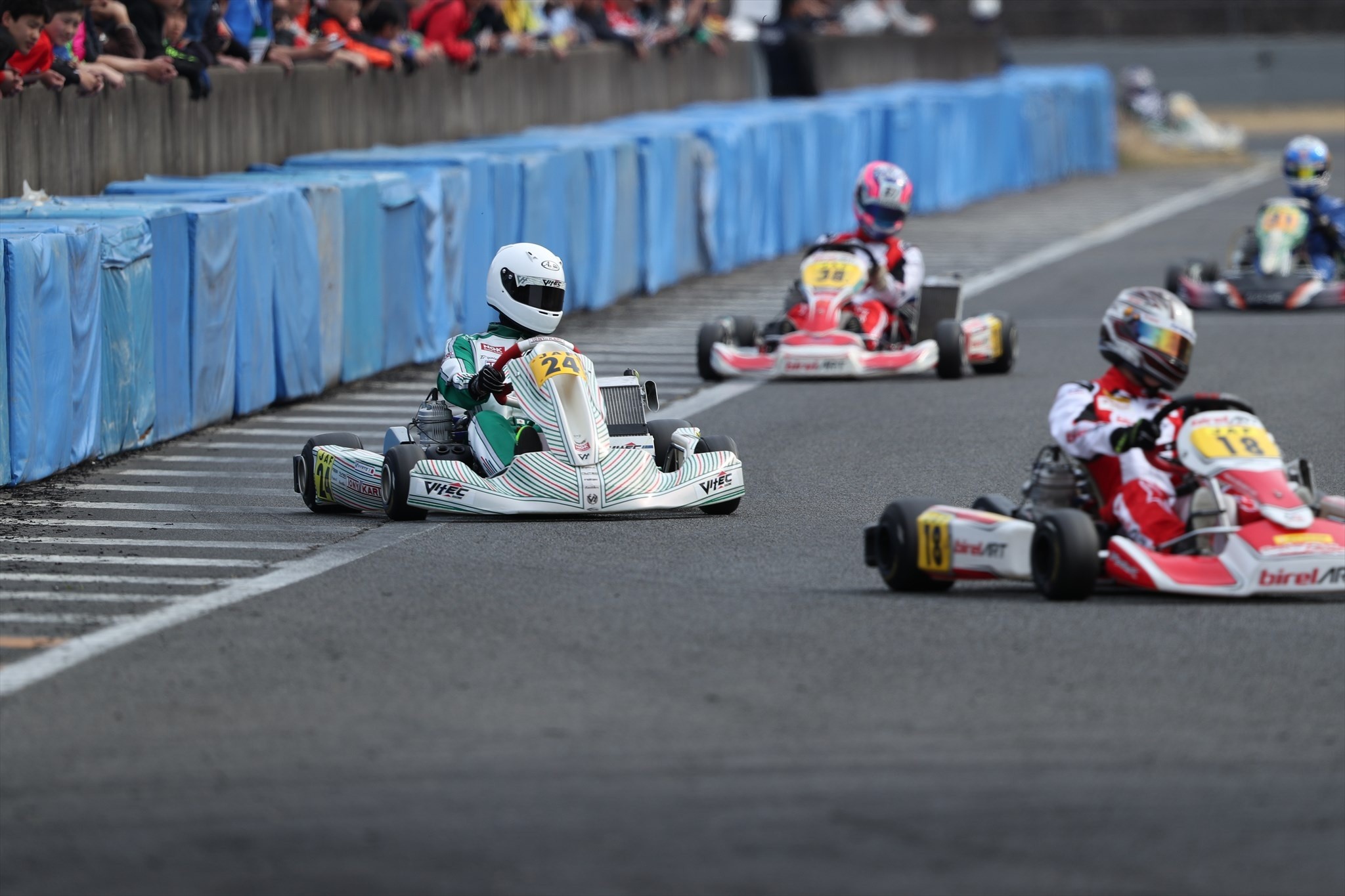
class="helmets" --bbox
[1283,135,1332,195]
[1099,286,1196,392]
[487,243,566,336]
[853,160,913,241]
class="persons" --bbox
[1242,135,1345,285]
[1122,66,1171,133]
[437,243,566,478]
[767,160,922,350]
[1049,287,1323,558]
[0,0,1019,99]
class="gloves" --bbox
[1112,419,1160,454]
[471,364,505,404]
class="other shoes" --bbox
[1174,486,1219,555]
[513,426,543,455]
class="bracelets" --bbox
[406,45,416,58]
